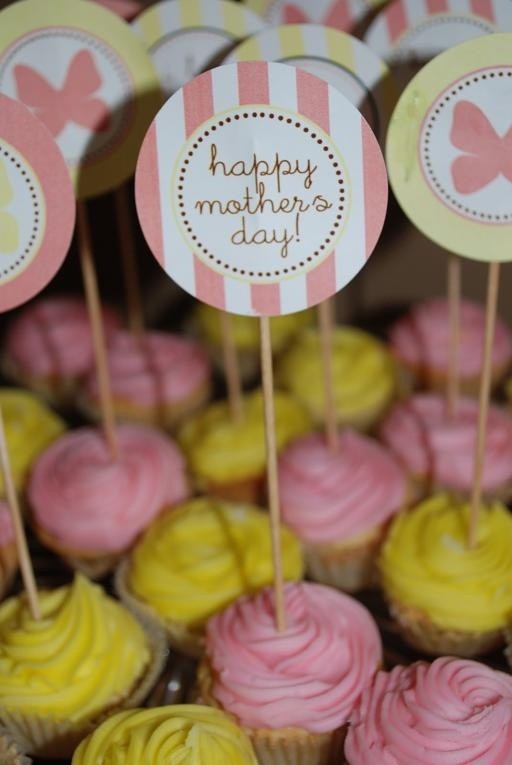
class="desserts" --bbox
[1,301,512,765]
